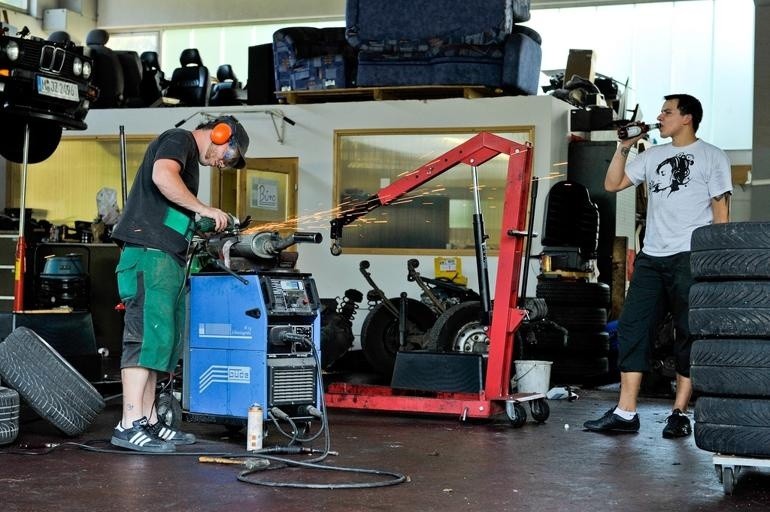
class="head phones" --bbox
[210,115,238,146]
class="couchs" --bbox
[273,0,542,104]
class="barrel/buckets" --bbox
[511,360,554,397]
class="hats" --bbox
[215,116,249,168]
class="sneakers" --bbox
[111,415,196,452]
[584,405,640,431]
[663,409,691,438]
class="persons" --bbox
[110,115,250,453]
[580,93,730,438]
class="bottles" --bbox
[617,122,663,141]
[245,403,264,453]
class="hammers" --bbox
[198,454,271,470]
